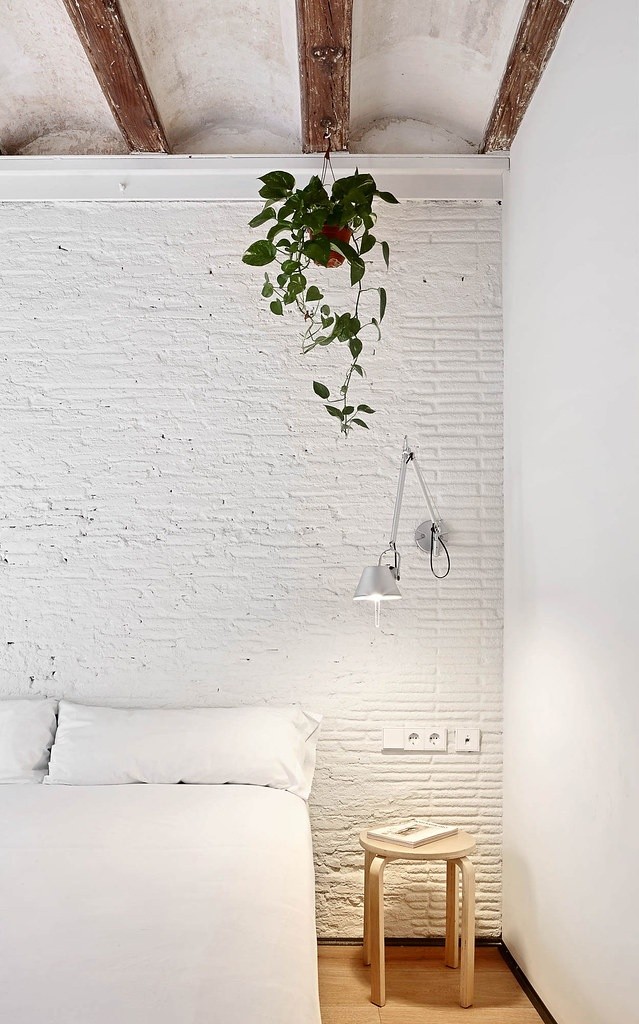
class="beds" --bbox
[0,752,323,1023]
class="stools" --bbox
[365,817,477,1009]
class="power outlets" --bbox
[407,728,423,749]
[455,727,481,751]
[427,726,449,750]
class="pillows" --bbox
[44,699,326,799]
[1,696,60,785]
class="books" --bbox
[367,819,458,849]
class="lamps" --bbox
[353,432,451,628]
